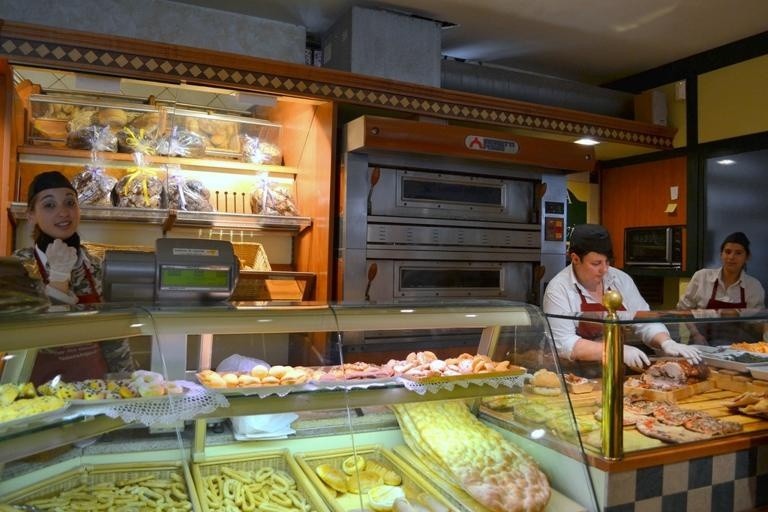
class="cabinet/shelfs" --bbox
[0,296,602,510]
[7,94,318,301]
[543,308,767,510]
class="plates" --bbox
[7,94,318,301]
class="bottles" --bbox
[29,171,77,200]
[724,232,750,246]
[570,225,611,254]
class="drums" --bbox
[649,355,693,361]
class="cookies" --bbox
[624,227,683,270]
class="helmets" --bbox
[311,374,399,385]
[0,400,71,426]
[701,346,768,382]
[58,385,191,404]
[199,378,307,392]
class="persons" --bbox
[13,172,139,386]
[675,233,767,346]
[537,223,702,374]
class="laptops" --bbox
[401,351,511,378]
[44,102,283,166]
[250,182,297,218]
[0,348,137,427]
[315,454,451,512]
[72,170,212,213]
[196,351,325,390]
[334,363,364,379]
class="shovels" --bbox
[388,399,551,512]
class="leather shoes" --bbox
[46,239,77,282]
[661,340,701,366]
[623,345,651,369]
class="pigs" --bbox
[130,369,182,397]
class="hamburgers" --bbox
[528,372,561,397]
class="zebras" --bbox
[336,149,567,354]
